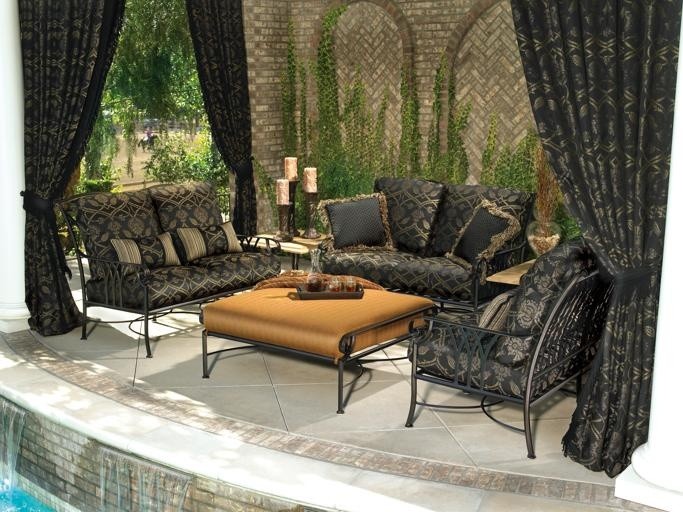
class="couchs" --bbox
[311,179,534,306]
[58,182,283,357]
[406,236,616,460]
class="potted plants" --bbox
[527,147,562,256]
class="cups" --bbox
[346,277,357,292]
[328,276,340,292]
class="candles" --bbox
[304,167,318,195]
[276,178,290,207]
[284,157,300,182]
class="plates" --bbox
[296,282,364,300]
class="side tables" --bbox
[237,230,333,270]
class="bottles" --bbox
[306,248,324,292]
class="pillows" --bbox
[176,220,244,262]
[109,231,182,277]
[447,199,520,277]
[486,258,537,286]
[320,192,391,254]
[477,286,519,331]
